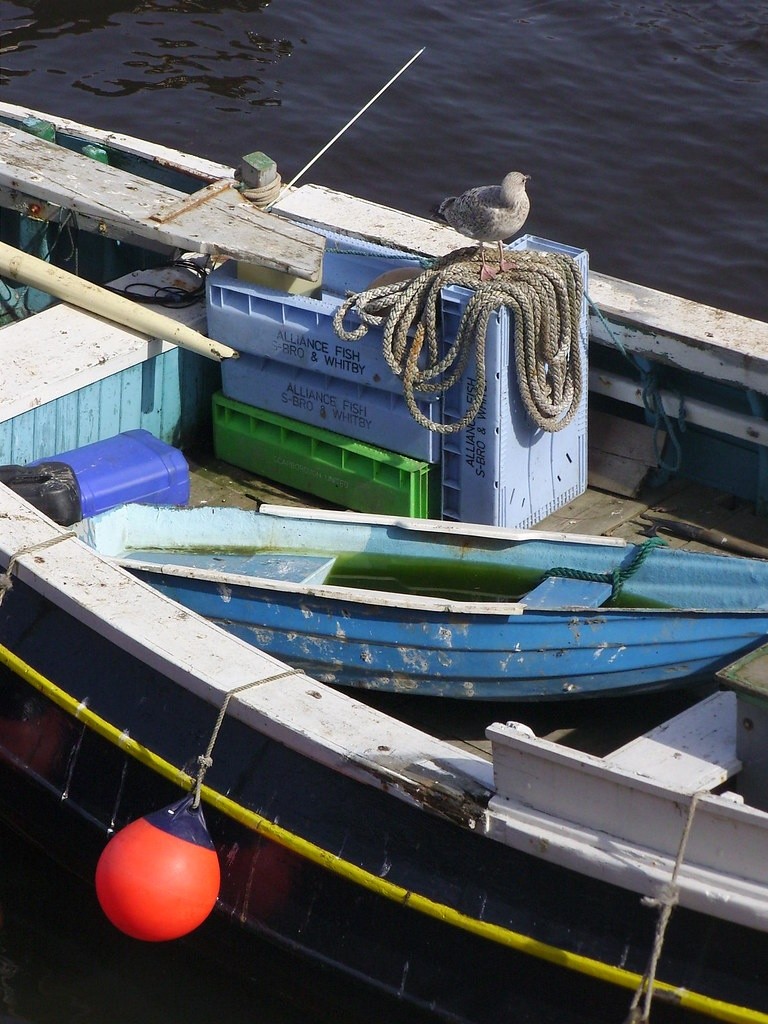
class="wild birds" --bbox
[428,171,532,283]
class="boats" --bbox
[1,95,768,1024]
[75,490,767,712]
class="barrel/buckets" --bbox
[25,428,190,525]
